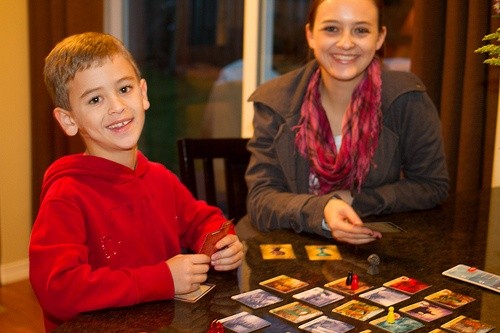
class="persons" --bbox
[28,32,243,333]
[234,0,451,245]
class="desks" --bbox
[53,185,500,333]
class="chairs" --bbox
[177,138,251,226]
